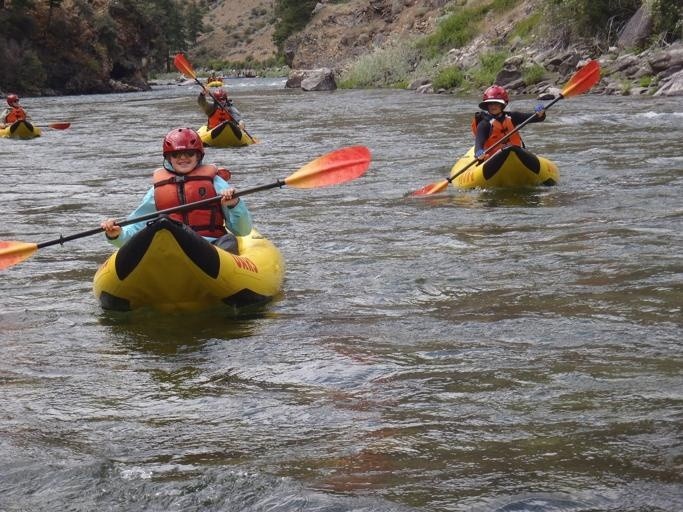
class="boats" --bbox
[0,119,41,140]
[447,142,562,193]
[195,118,252,149]
[88,216,286,313]
[204,80,227,88]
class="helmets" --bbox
[214,90,227,103]
[483,85,508,102]
[163,128,203,160]
[7,95,19,104]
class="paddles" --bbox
[173,53,257,146]
[4,122,69,130]
[0,146,370,268]
[412,61,601,196]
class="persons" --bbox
[2,92,28,128]
[474,85,546,163]
[197,88,240,130]
[99,123,252,256]
